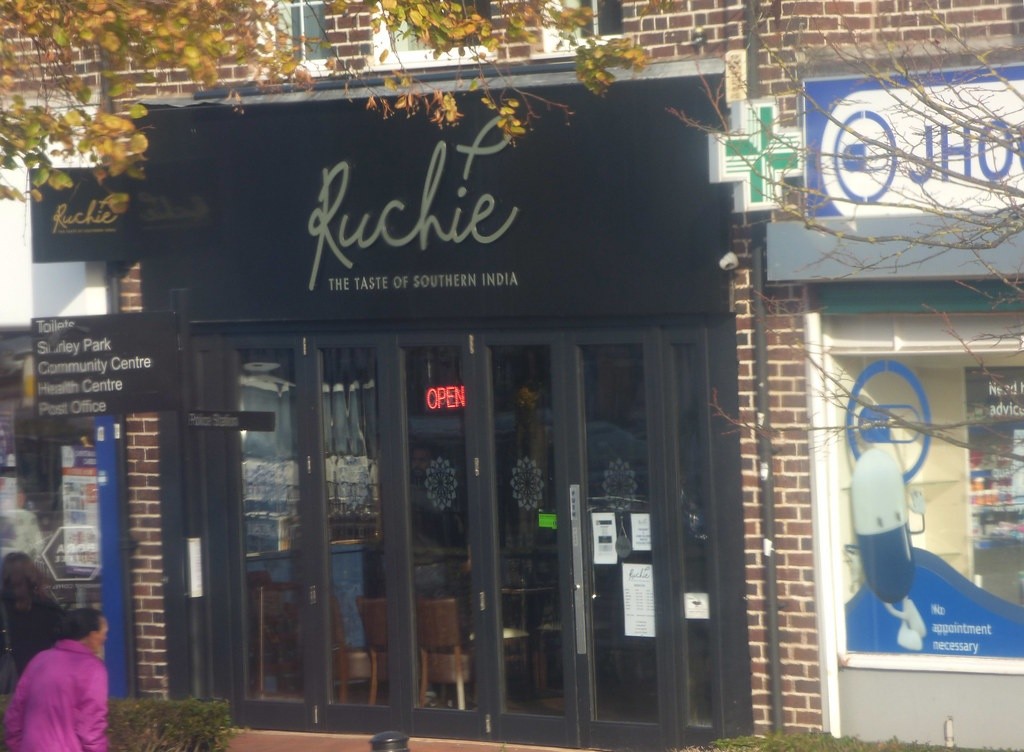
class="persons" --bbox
[0,607,114,752]
[0,552,64,694]
[407,444,474,708]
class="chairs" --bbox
[356,547,648,711]
[245,570,372,703]
